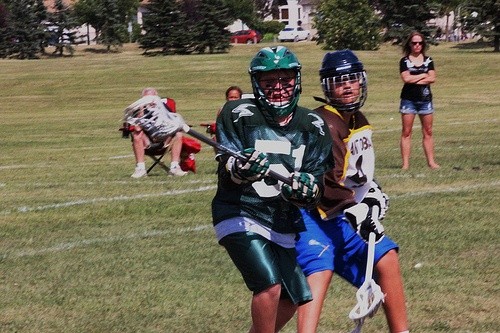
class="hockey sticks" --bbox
[348,206,386,333]
[118,95,294,187]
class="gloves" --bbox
[360,178,390,220]
[281,171,321,205]
[231,147,271,183]
[343,203,385,245]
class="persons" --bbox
[218,85,242,117]
[295,49,408,333]
[212,46,333,333]
[130,88,188,178]
[399,32,441,170]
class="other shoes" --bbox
[168,166,190,177]
[129,169,148,179]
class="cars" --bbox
[277,26,311,42]
[229,29,262,44]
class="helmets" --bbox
[247,46,302,118]
[319,50,368,113]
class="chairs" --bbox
[129,97,176,175]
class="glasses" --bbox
[409,41,423,45]
